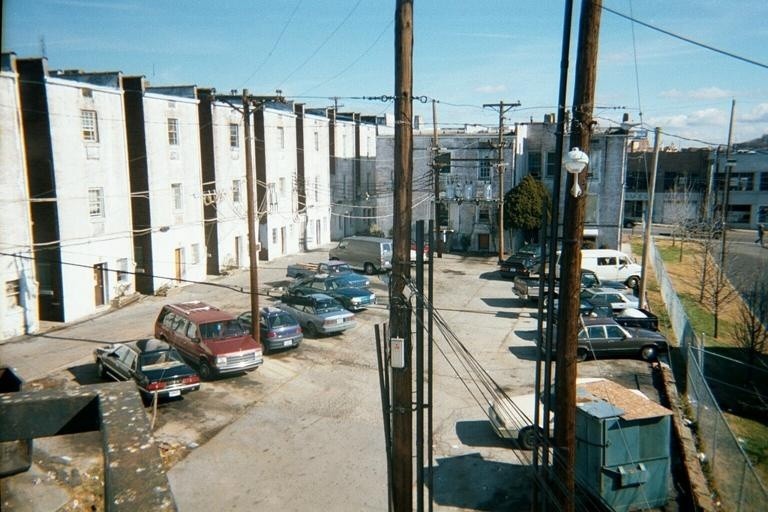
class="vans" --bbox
[329,237,396,274]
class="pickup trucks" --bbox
[286,261,370,288]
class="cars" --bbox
[681,217,722,239]
[485,239,673,451]
[624,216,636,228]
[233,307,305,354]
[285,276,377,313]
[96,335,200,404]
[409,240,430,263]
[272,292,356,335]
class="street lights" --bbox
[618,119,664,310]
[550,144,590,511]
[719,157,738,287]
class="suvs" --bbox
[157,302,263,375]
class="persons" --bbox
[754,222,765,245]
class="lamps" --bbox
[563,147,590,198]
